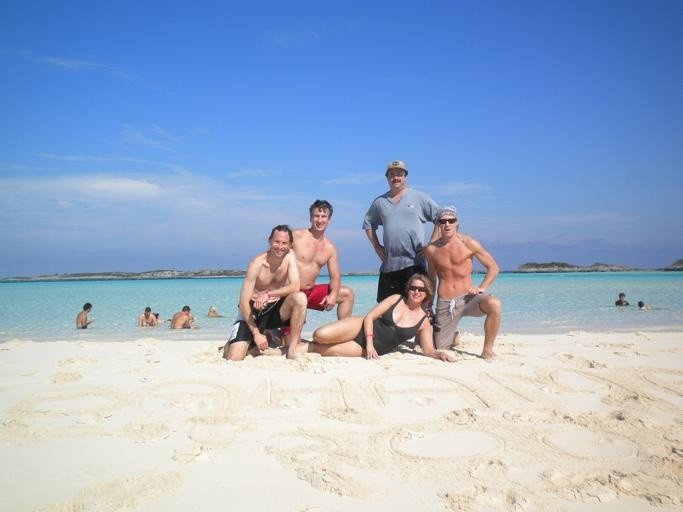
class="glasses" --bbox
[438,218,456,224]
[409,285,426,292]
[389,172,403,177]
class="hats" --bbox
[385,160,408,175]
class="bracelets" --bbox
[366,334,375,337]
[265,291,271,299]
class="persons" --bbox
[638,300,646,310]
[208,305,220,317]
[615,293,629,306]
[361,159,445,303]
[424,209,501,359]
[281,198,355,347]
[76,303,95,329]
[138,305,194,330]
[222,224,308,362]
[313,272,457,362]
